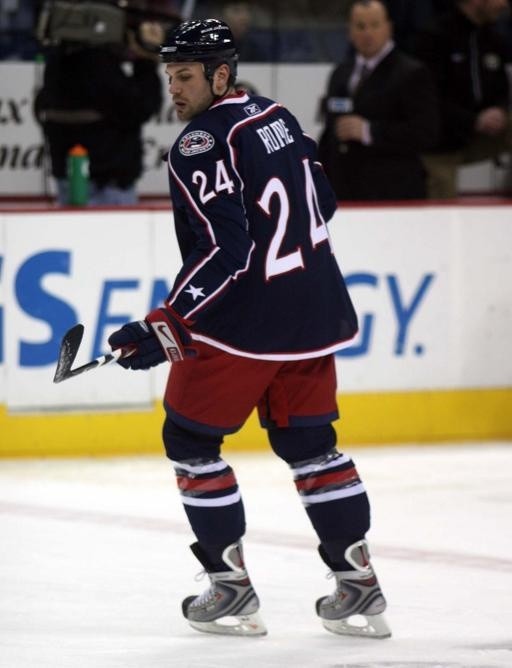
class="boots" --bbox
[182,541,260,623]
[316,537,387,622]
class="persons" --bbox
[317,0,512,206]
[205,1,255,64]
[2,1,181,206]
[107,17,388,622]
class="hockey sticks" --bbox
[53,324,138,384]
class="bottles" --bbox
[65,145,92,206]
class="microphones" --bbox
[326,81,356,155]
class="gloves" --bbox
[107,302,196,371]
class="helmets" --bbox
[159,16,239,84]
